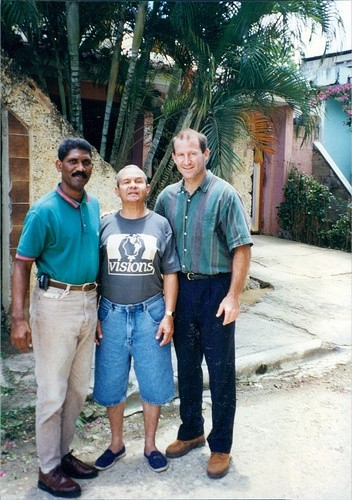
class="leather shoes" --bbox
[37,468,82,499]
[61,452,97,480]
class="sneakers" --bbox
[142,448,169,473]
[206,449,231,479]
[94,442,126,470]
[165,433,206,459]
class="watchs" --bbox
[165,310,176,319]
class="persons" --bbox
[151,128,254,479]
[12,139,99,499]
[92,165,181,473]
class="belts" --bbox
[48,281,101,292]
[178,271,210,280]
